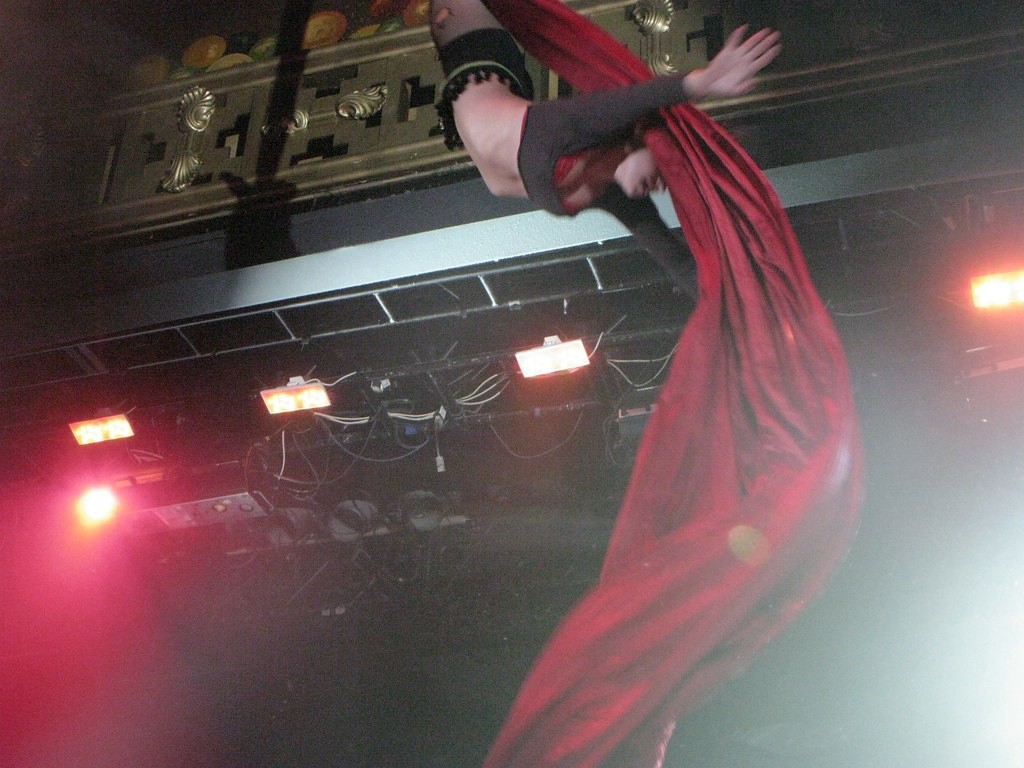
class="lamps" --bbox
[254,488,448,547]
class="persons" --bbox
[428,0,785,300]
